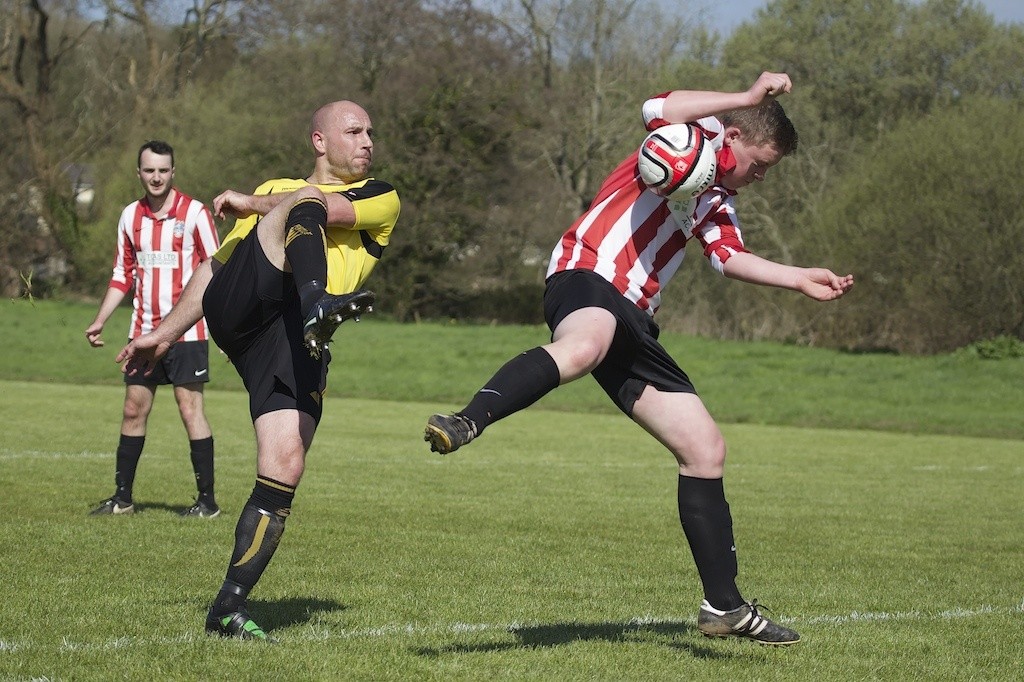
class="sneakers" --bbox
[205,605,279,644]
[698,599,801,647]
[182,500,221,518]
[90,495,134,515]
[303,289,375,361]
[424,413,477,455]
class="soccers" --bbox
[638,124,717,203]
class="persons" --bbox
[84,140,221,520]
[113,98,403,645]
[415,70,856,646]
